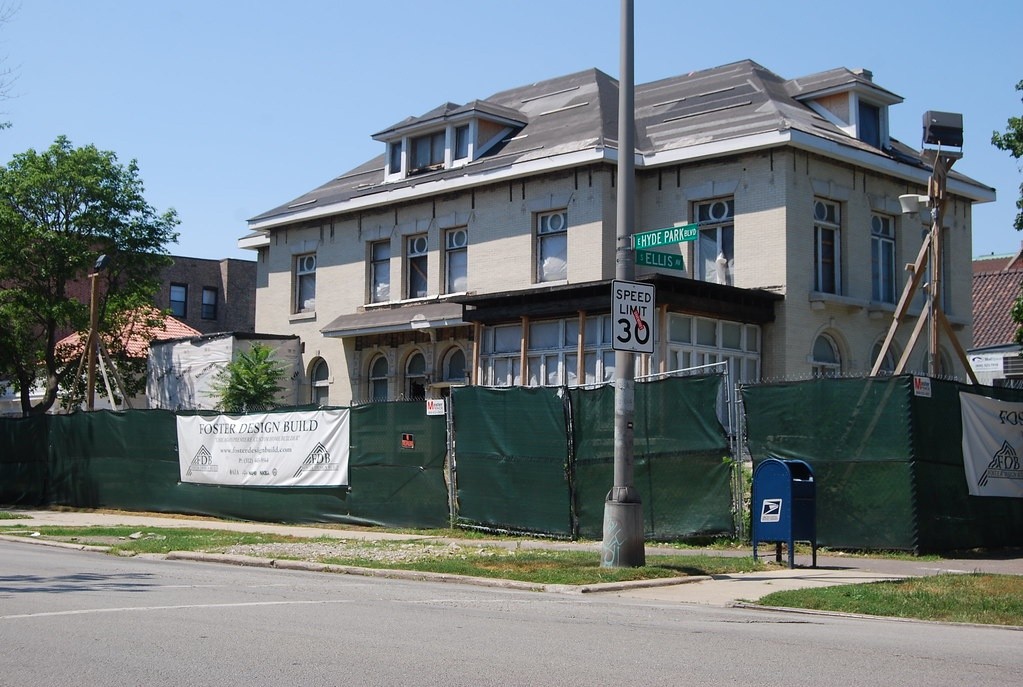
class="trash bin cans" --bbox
[750,459,816,543]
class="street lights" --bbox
[86,254,111,414]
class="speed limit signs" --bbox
[611,281,653,354]
